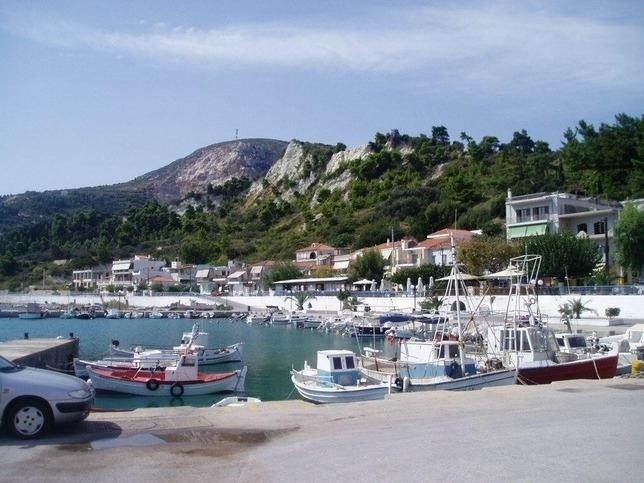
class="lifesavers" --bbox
[170,383,184,396]
[147,379,159,391]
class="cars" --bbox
[211,289,225,296]
[0,354,96,439]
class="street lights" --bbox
[600,215,609,273]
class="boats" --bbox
[110,321,245,366]
[18,303,271,325]
[271,310,289,326]
[72,358,248,397]
